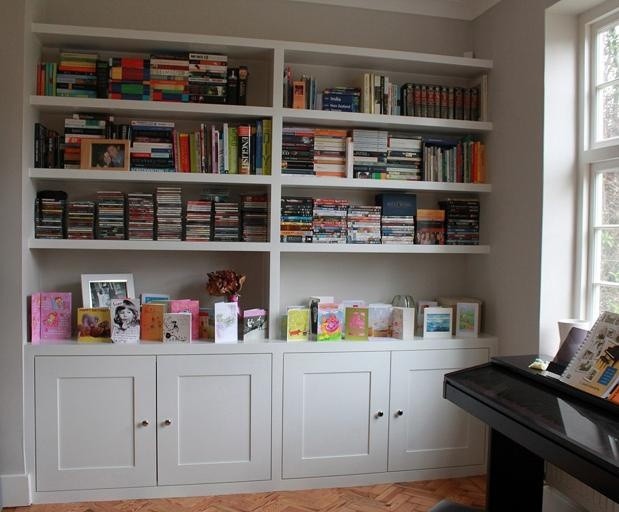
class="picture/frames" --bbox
[80,137,131,172]
[109,298,141,342]
[456,303,480,338]
[421,307,453,340]
[141,292,170,309]
[416,299,437,327]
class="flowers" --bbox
[205,269,246,296]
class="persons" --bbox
[107,144,124,167]
[103,284,119,299]
[96,151,114,167]
[97,289,110,307]
[114,300,140,330]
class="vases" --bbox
[220,293,244,322]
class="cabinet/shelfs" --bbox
[23,351,276,494]
[287,349,492,481]
[25,30,502,345]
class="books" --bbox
[283,66,489,122]
[35,51,249,106]
[131,112,272,175]
[559,309,619,398]
[65,112,131,144]
[280,127,486,184]
[280,194,480,246]
[34,123,80,168]
[35,185,268,241]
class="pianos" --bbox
[443,352,619,509]
